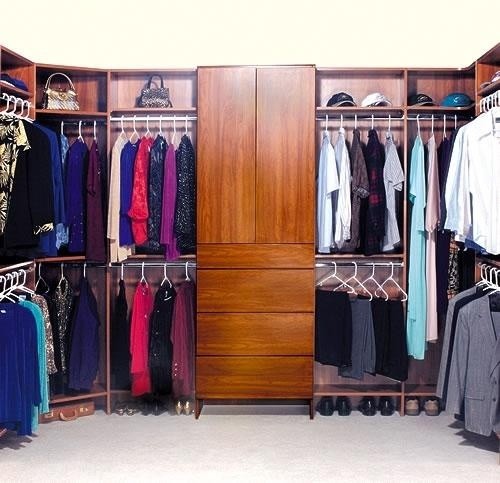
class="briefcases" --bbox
[38,400,95,424]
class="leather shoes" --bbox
[113,400,194,416]
[315,396,394,416]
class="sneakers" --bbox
[406,397,439,415]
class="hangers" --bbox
[1,258,193,303]
[322,90,500,139]
[321,259,500,304]
[0,91,192,147]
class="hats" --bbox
[440,93,471,107]
[408,95,433,106]
[327,92,355,107]
[362,93,392,106]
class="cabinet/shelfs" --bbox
[317,40,500,439]
[195,62,319,422]
[1,43,198,441]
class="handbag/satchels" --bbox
[42,73,79,110]
[138,74,169,108]
